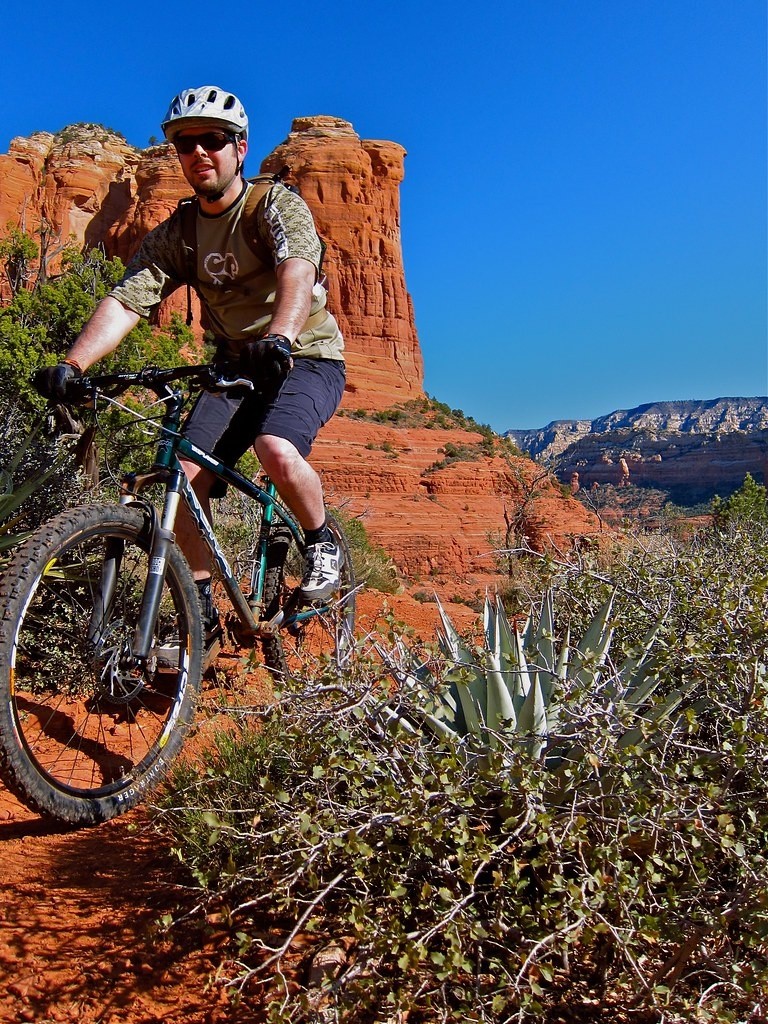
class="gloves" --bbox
[237,334,294,395]
[33,358,85,403]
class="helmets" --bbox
[160,84,249,143]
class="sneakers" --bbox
[299,525,345,601]
[155,607,221,669]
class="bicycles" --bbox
[0,354,358,827]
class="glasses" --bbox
[172,132,238,154]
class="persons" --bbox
[578,536,593,554]
[36,85,345,669]
